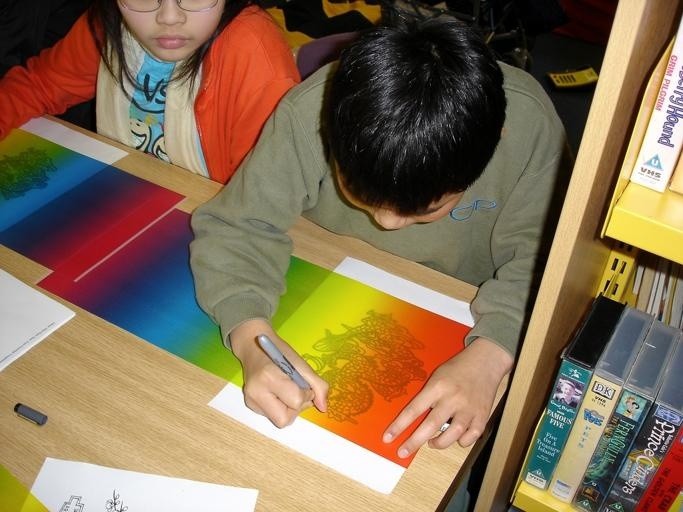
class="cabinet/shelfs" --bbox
[475,0,683,512]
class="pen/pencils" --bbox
[257,335,311,390]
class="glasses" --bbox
[120,1,220,13]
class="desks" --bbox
[0,112,514,512]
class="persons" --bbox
[0,0,304,188]
[187,13,576,460]
